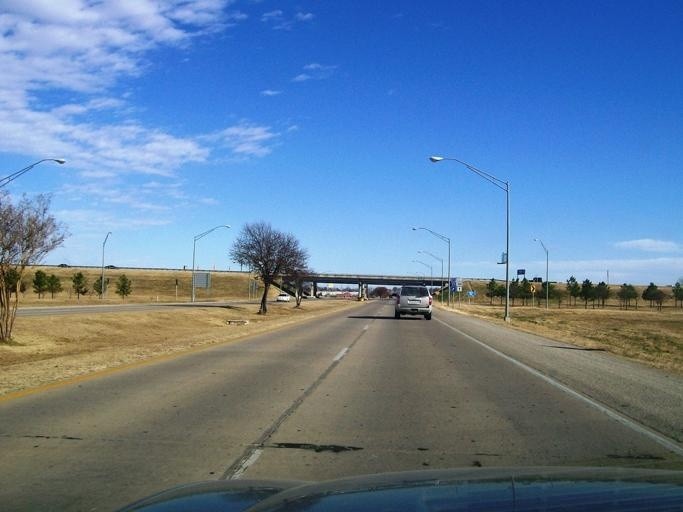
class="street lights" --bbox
[190,224,230,304]
[100,231,112,299]
[428,155,512,324]
[533,237,549,310]
[410,226,451,308]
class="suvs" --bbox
[393,283,433,320]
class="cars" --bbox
[276,293,290,303]
